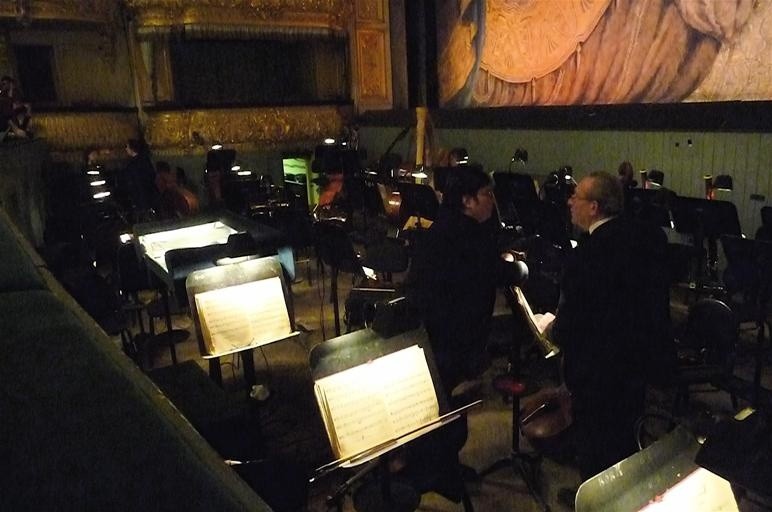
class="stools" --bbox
[478,373,553,512]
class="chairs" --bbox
[660,298,739,433]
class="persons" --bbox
[0,77,161,210]
[448,148,468,167]
[420,170,530,481]
[543,170,645,510]
